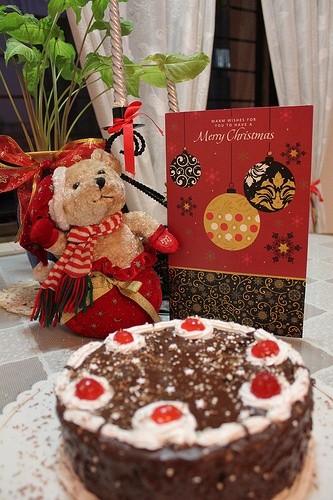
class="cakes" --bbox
[50,316,316,500]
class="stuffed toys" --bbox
[22,147,179,342]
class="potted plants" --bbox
[1,1,211,286]
